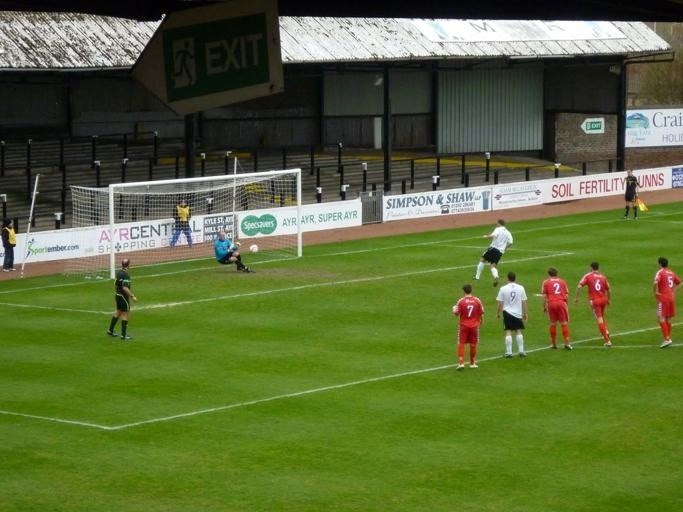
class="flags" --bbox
[636,196,648,213]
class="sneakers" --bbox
[624,216,638,220]
[660,339,672,348]
[473,275,499,286]
[3,268,16,272]
[456,364,478,370]
[504,352,526,358]
[551,345,572,350]
[107,329,132,339]
[237,266,252,273]
[603,330,612,346]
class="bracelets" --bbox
[131,295,134,297]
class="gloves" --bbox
[227,242,241,252]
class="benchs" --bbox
[1,135,584,236]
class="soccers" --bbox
[249,244,258,254]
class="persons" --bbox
[472,221,513,286]
[653,257,683,348]
[214,232,254,272]
[573,261,612,346]
[541,267,573,350]
[495,271,528,358]
[452,286,485,369]
[107,259,136,340]
[2,219,16,272]
[170,199,192,247]
[622,169,638,219]
